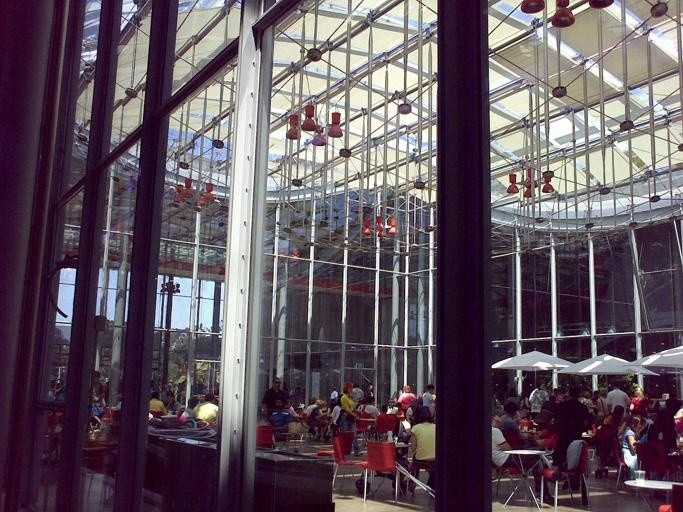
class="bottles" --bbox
[518,417,537,433]
[387,430,398,446]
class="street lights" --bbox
[159,281,180,388]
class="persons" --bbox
[46,368,219,424]
[287,377,436,495]
[260,376,287,416]
[489,377,682,505]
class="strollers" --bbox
[354,441,412,495]
[591,424,630,481]
[266,408,301,450]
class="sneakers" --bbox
[353,450,363,457]
[538,493,560,506]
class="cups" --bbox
[634,470,644,483]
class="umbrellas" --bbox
[490,349,576,390]
[550,350,659,395]
[620,345,682,375]
